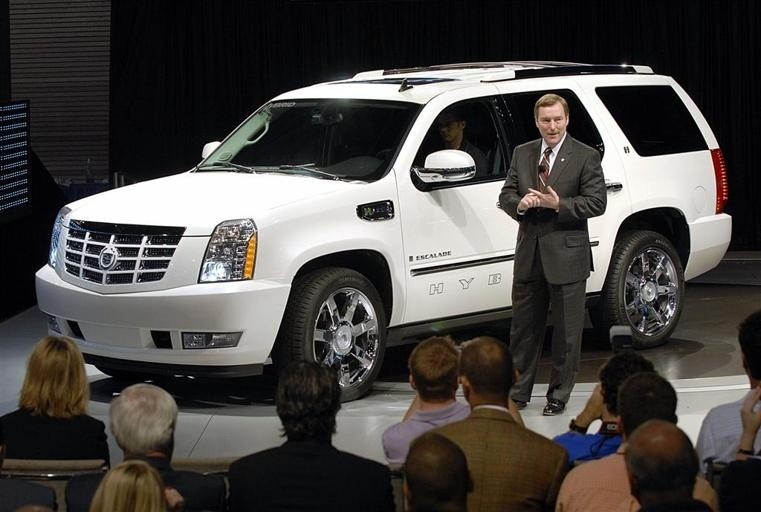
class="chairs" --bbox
[170,458,238,512]
[467,122,502,178]
[703,454,729,487]
[0,458,107,511]
[390,463,409,511]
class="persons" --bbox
[552,350,719,511]
[429,335,571,511]
[108,382,225,512]
[380,336,470,467]
[0,335,110,512]
[402,433,474,512]
[694,310,761,472]
[225,361,397,512]
[91,460,168,512]
[438,111,492,176]
[498,93,607,415]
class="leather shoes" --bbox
[543,399,565,416]
[514,399,527,407]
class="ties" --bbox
[538,148,552,191]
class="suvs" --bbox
[35,61,733,404]
